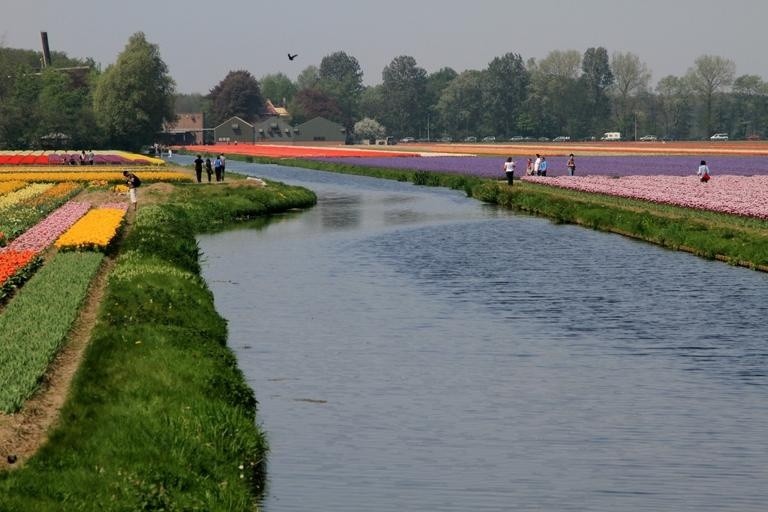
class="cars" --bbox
[710,133,728,141]
[509,136,571,142]
[400,136,496,143]
[745,135,760,141]
[639,135,659,141]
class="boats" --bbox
[147,147,175,156]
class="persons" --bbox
[533,154,541,176]
[234,140,238,146]
[536,157,547,176]
[122,171,137,211]
[203,158,212,185]
[503,157,517,186]
[566,154,576,176]
[69,154,74,167]
[88,148,95,167]
[525,159,535,176]
[194,154,203,183]
[219,153,226,181]
[697,161,710,183]
[62,156,67,166]
[213,156,222,182]
[80,149,86,167]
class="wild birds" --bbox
[287,53,298,60]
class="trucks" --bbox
[601,132,621,142]
